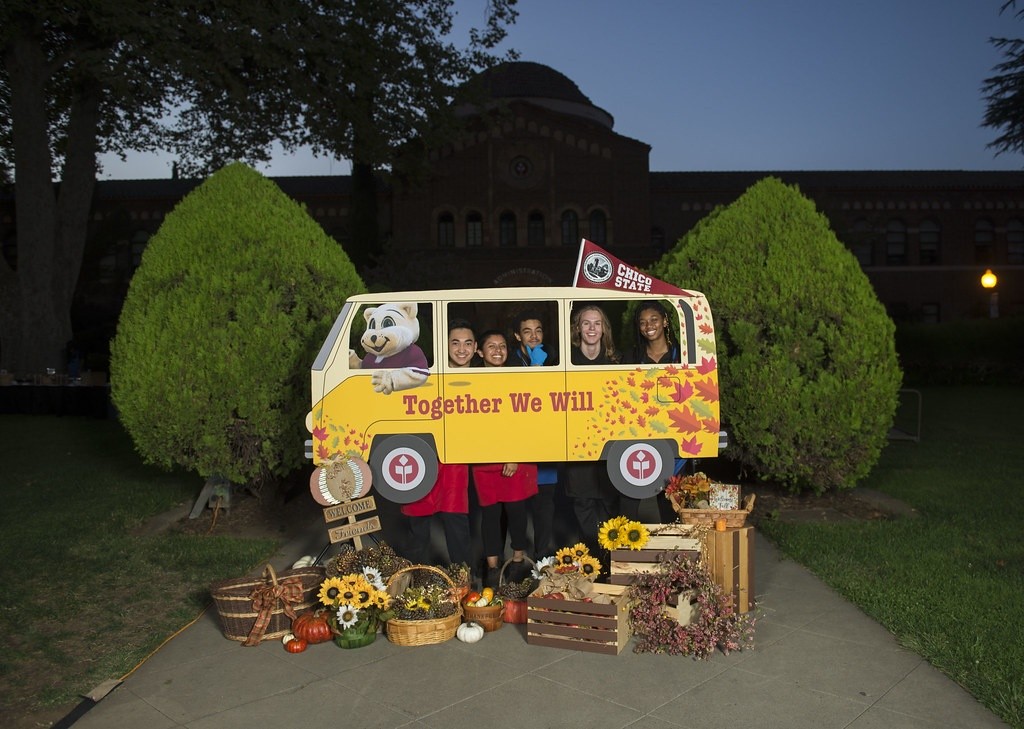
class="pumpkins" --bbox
[293,555,319,568]
[457,589,527,644]
[283,611,334,652]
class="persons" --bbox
[399,317,561,590]
[566,302,690,581]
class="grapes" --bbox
[392,599,457,620]
[500,582,534,598]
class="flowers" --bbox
[628,546,766,662]
[598,515,652,551]
[531,542,602,582]
[317,544,470,630]
[665,472,722,508]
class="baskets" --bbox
[210,563,325,641]
[385,564,463,644]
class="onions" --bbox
[534,593,608,630]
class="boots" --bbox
[485,568,500,588]
[507,559,524,582]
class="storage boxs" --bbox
[527,579,634,655]
[612,524,704,629]
[705,525,754,615]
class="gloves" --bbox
[526,344,547,366]
